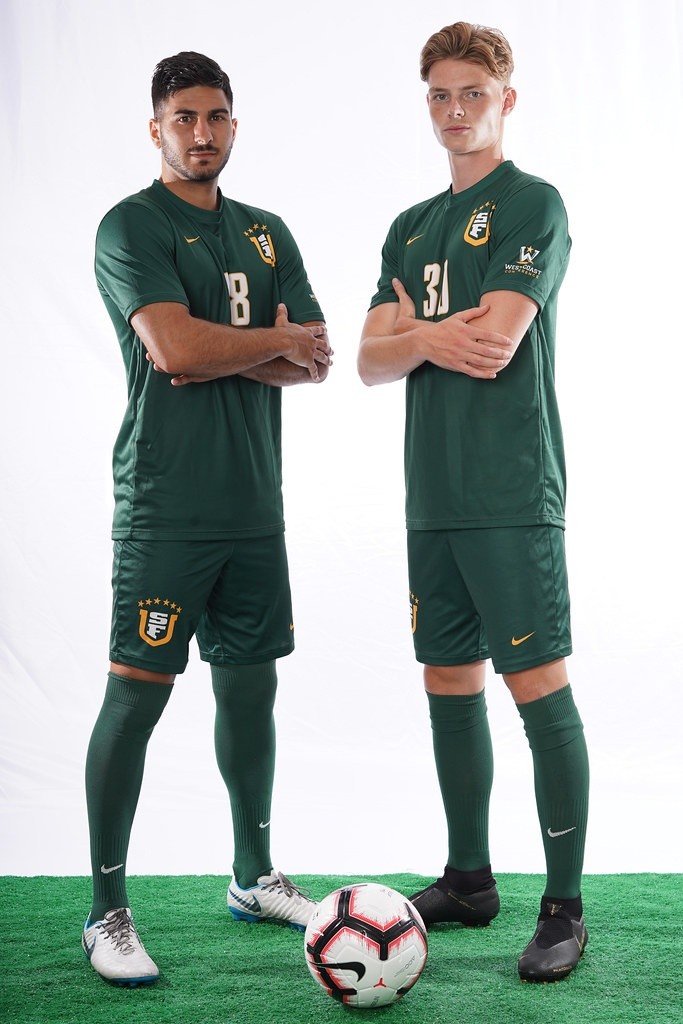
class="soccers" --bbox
[304,882,428,1008]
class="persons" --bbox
[355,19,592,982]
[80,50,320,987]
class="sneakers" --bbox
[226,870,320,932]
[517,902,587,985]
[81,908,159,987]
[406,875,499,927]
